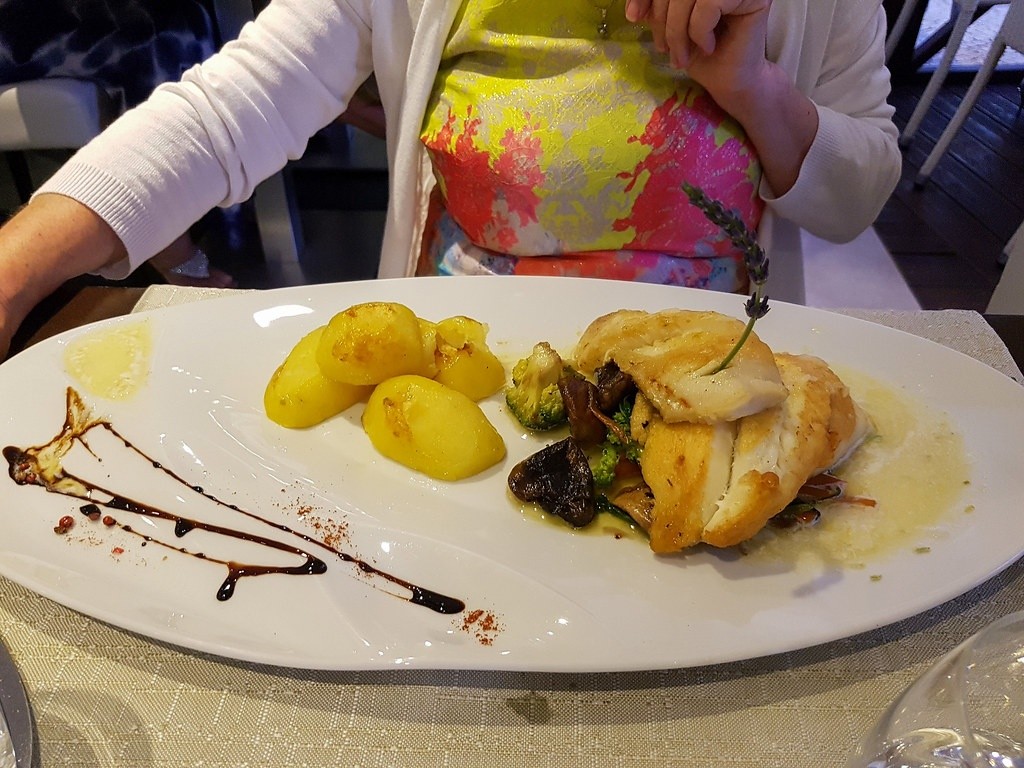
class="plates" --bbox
[0,276,1024,673]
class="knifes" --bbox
[0,637,32,768]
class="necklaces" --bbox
[590,0,614,35]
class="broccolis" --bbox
[505,340,648,485]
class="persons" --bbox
[0,0,902,365]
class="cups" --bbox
[848,609,1024,767]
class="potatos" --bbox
[262,301,509,482]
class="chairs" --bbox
[0,77,160,348]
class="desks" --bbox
[0,284,1024,767]
